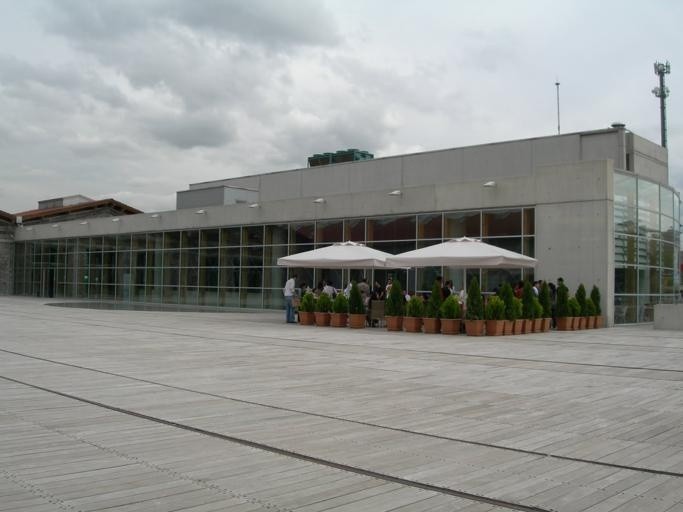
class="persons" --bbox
[402,275,455,304]
[282,274,393,323]
[492,277,567,303]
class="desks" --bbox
[644,306,653,321]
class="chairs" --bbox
[614,304,628,323]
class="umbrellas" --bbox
[385,236,538,304]
[277,240,412,285]
